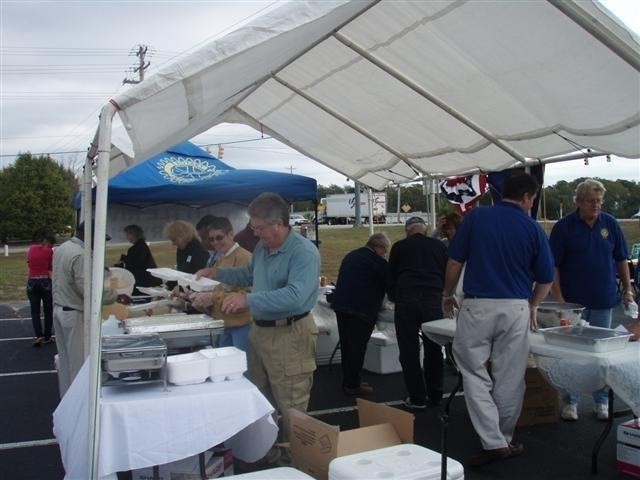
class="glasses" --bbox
[247,224,256,230]
[585,198,605,205]
[208,234,225,241]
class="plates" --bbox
[165,347,248,385]
[538,324,635,353]
[146,268,220,292]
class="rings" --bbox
[231,304,234,308]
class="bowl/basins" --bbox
[533,302,586,326]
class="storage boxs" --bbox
[288,397,414,480]
[616,419,640,477]
[364,330,423,375]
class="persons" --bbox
[388,216,449,409]
[446,172,556,468]
[247,191,320,467]
[331,232,390,396]
[195,214,222,267]
[51,219,135,400]
[113,224,164,296]
[193,217,253,381]
[27,234,56,346]
[164,220,210,291]
[550,180,635,421]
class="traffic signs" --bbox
[401,203,412,213]
[317,203,326,211]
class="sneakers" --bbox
[43,336,55,344]
[344,385,373,394]
[592,402,608,420]
[466,446,510,465]
[508,442,523,456]
[361,382,368,386]
[560,402,578,421]
[32,337,42,346]
[402,396,426,411]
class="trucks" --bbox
[319,193,387,226]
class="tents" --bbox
[87,1,640,479]
[76,141,319,256]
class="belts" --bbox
[255,311,308,328]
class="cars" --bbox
[289,215,311,226]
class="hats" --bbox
[405,217,425,225]
[79,218,112,240]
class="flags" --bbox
[441,174,487,216]
